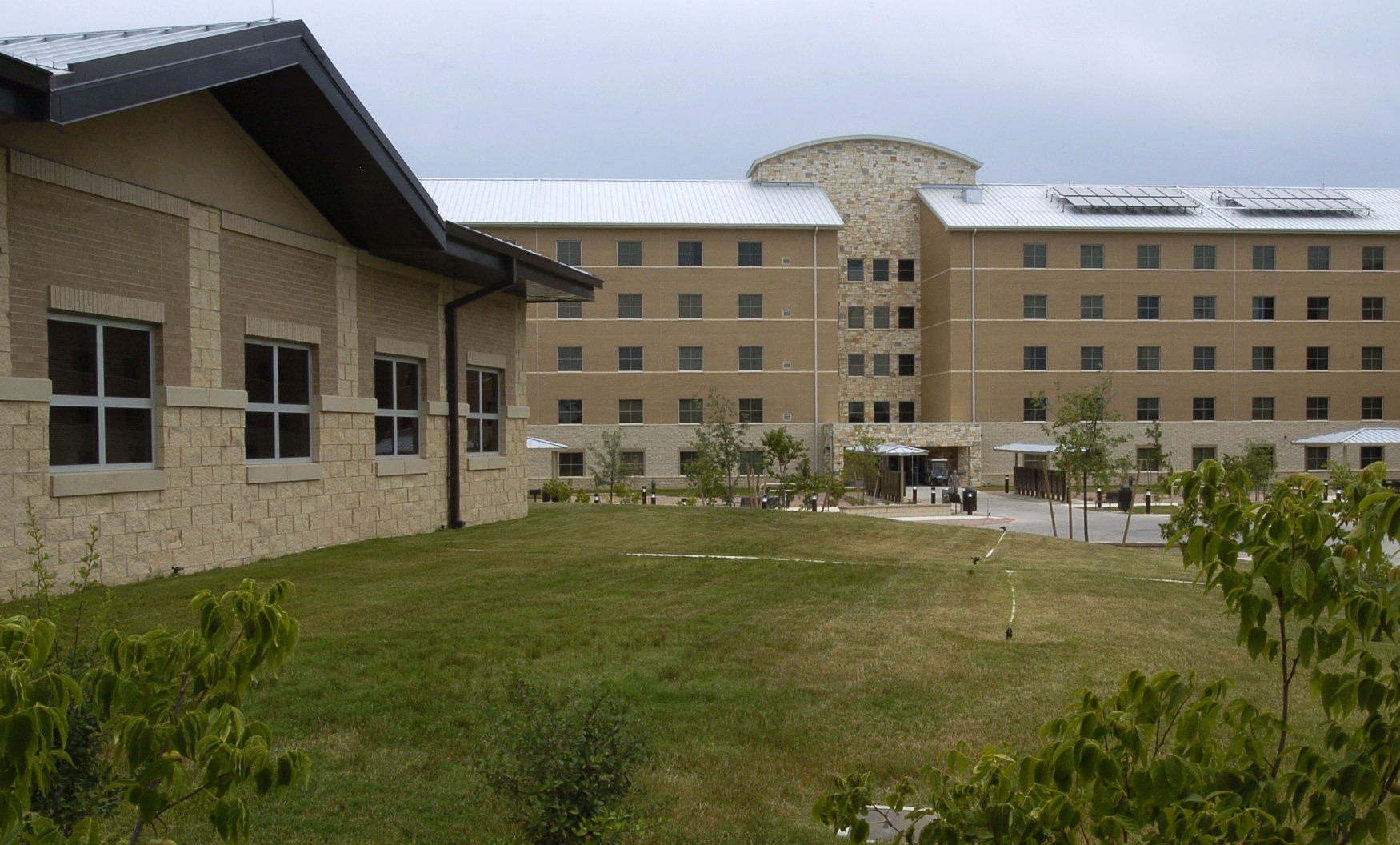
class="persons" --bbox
[947,470,960,494]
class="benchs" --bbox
[1095,492,1118,512]
[734,497,780,509]
[945,493,962,514]
